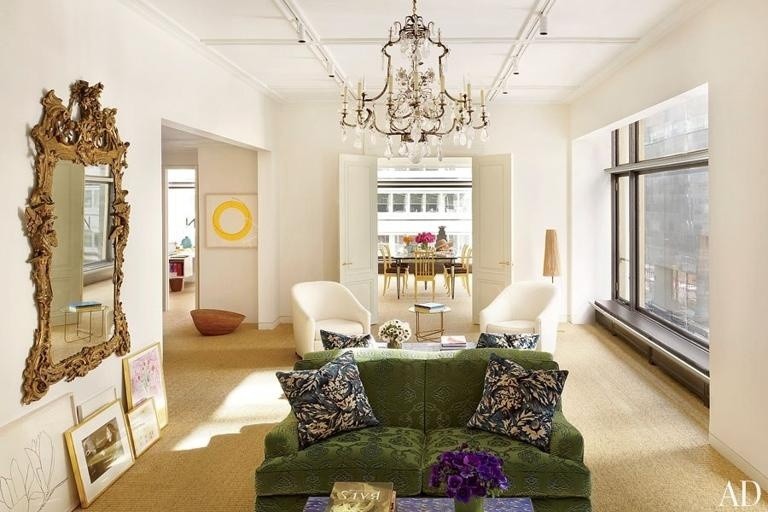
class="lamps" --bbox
[498,10,547,96]
[289,19,336,79]
[335,0,492,171]
[542,227,563,285]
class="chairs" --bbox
[375,243,472,303]
[290,281,372,359]
[478,280,558,356]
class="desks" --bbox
[409,306,451,342]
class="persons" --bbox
[83,423,117,483]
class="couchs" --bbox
[253,351,590,511]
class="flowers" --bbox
[425,442,509,498]
[377,319,411,343]
[402,234,415,242]
[415,231,436,249]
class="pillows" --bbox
[272,352,379,451]
[467,351,569,453]
[316,328,373,352]
[473,333,541,350]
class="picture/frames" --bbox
[121,342,170,430]
[62,399,136,507]
[123,396,162,460]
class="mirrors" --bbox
[20,80,134,407]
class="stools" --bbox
[62,301,107,341]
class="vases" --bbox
[386,343,403,350]
[456,493,483,512]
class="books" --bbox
[415,302,445,313]
[440,335,467,351]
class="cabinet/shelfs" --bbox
[170,256,194,281]
